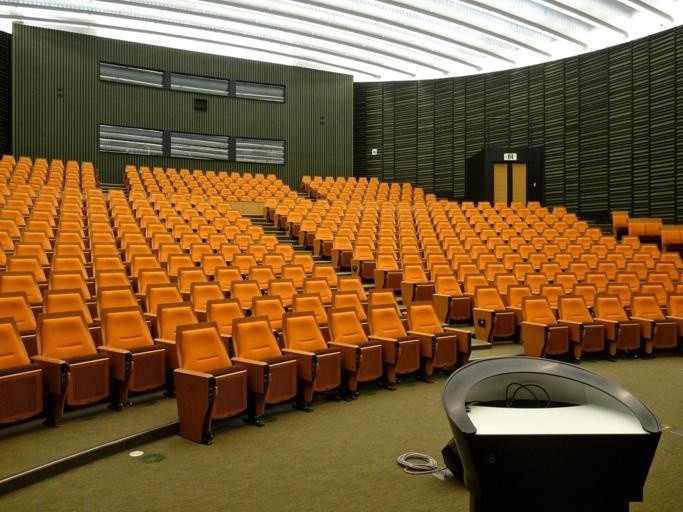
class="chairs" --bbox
[0,154,683,447]
